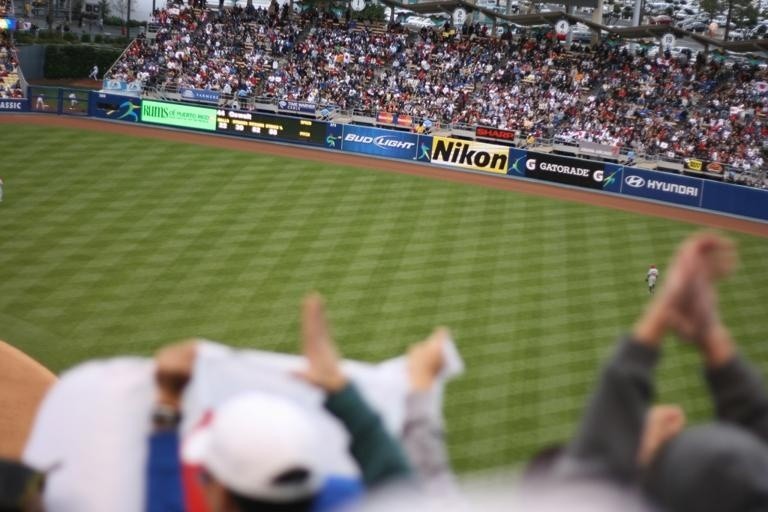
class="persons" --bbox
[644,263,660,294]
[2,2,767,190]
[143,289,412,512]
[1,327,768,510]
[554,227,767,510]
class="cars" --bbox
[479,1,768,44]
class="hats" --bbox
[176,389,355,507]
